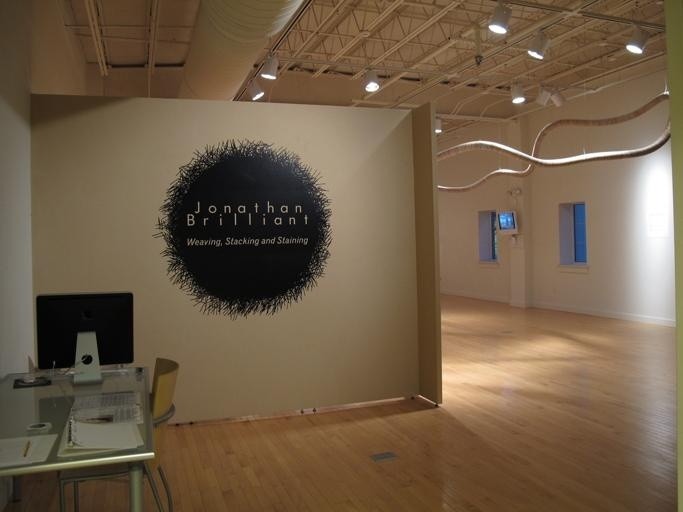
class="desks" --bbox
[0,364,156,512]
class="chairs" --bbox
[58,358,180,512]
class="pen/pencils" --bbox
[23,441,30,457]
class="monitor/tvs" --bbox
[498,210,519,235]
[34,291,134,386]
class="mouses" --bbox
[136,366,145,372]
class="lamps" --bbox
[487,1,513,34]
[435,115,442,133]
[526,28,552,59]
[535,83,566,108]
[260,52,279,80]
[510,79,526,104]
[363,68,381,93]
[245,81,264,101]
[626,24,650,55]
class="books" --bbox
[58,392,145,458]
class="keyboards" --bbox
[64,367,128,374]
